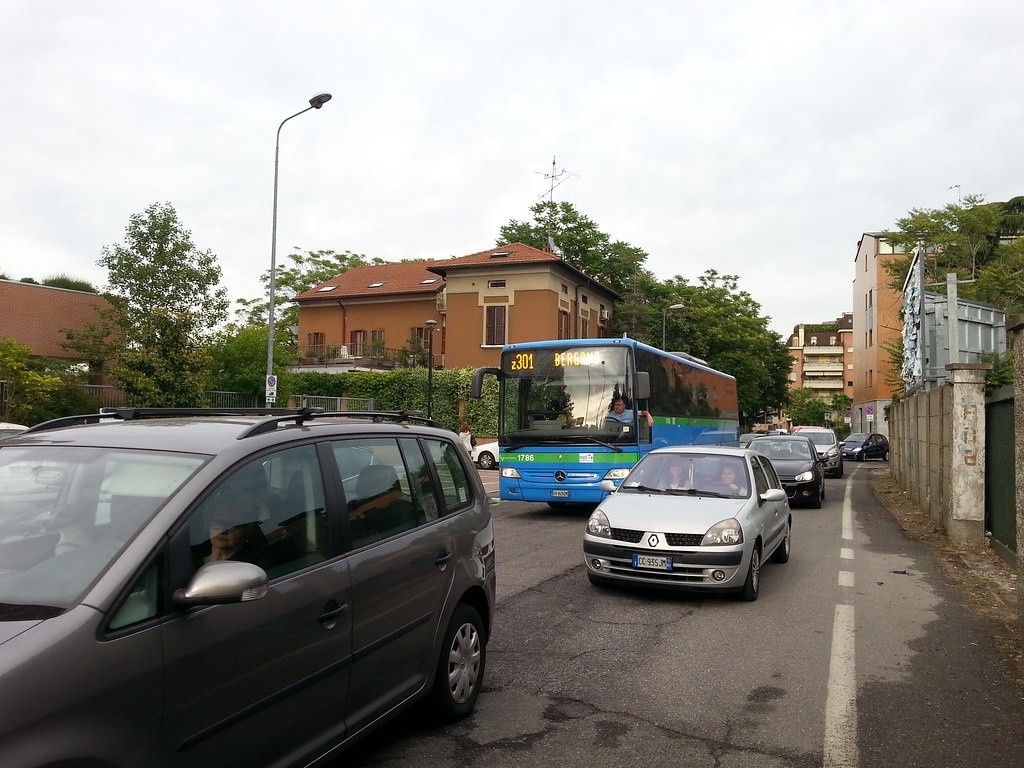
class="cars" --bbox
[743,436,830,509]
[582,444,793,601]
[470,440,499,470]
[839,433,889,462]
[694,431,739,451]
[0,420,30,443]
[736,428,790,457]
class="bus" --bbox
[467,337,741,509]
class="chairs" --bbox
[346,465,419,553]
[261,471,308,570]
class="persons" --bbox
[708,463,747,497]
[199,498,253,563]
[605,395,654,427]
[655,457,694,490]
[458,424,472,458]
[758,443,811,459]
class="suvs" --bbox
[788,427,847,479]
[0,407,495,768]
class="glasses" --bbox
[210,518,242,532]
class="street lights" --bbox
[264,92,336,409]
[426,318,437,424]
[662,303,684,351]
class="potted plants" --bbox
[285,345,344,366]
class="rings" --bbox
[651,421,653,423]
